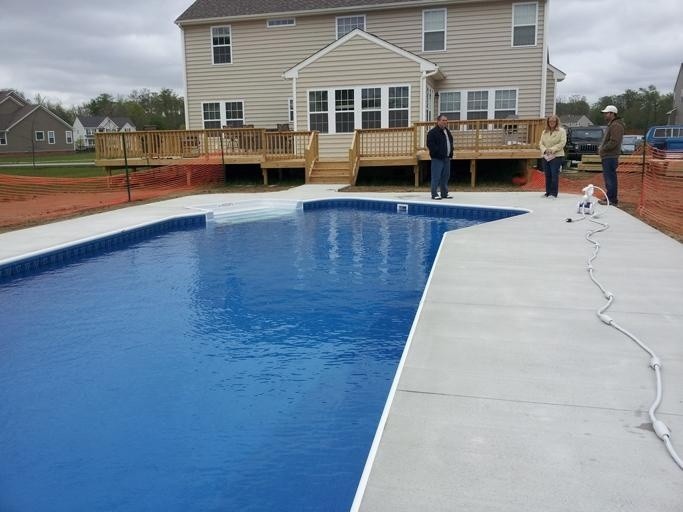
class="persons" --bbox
[425,114,455,200]
[538,114,567,198]
[597,105,624,206]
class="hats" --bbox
[600,105,618,113]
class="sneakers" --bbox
[600,198,618,206]
[598,195,607,203]
[547,192,557,198]
[540,193,549,198]
[434,196,442,200]
[442,195,453,199]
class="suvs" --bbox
[562,126,682,159]
